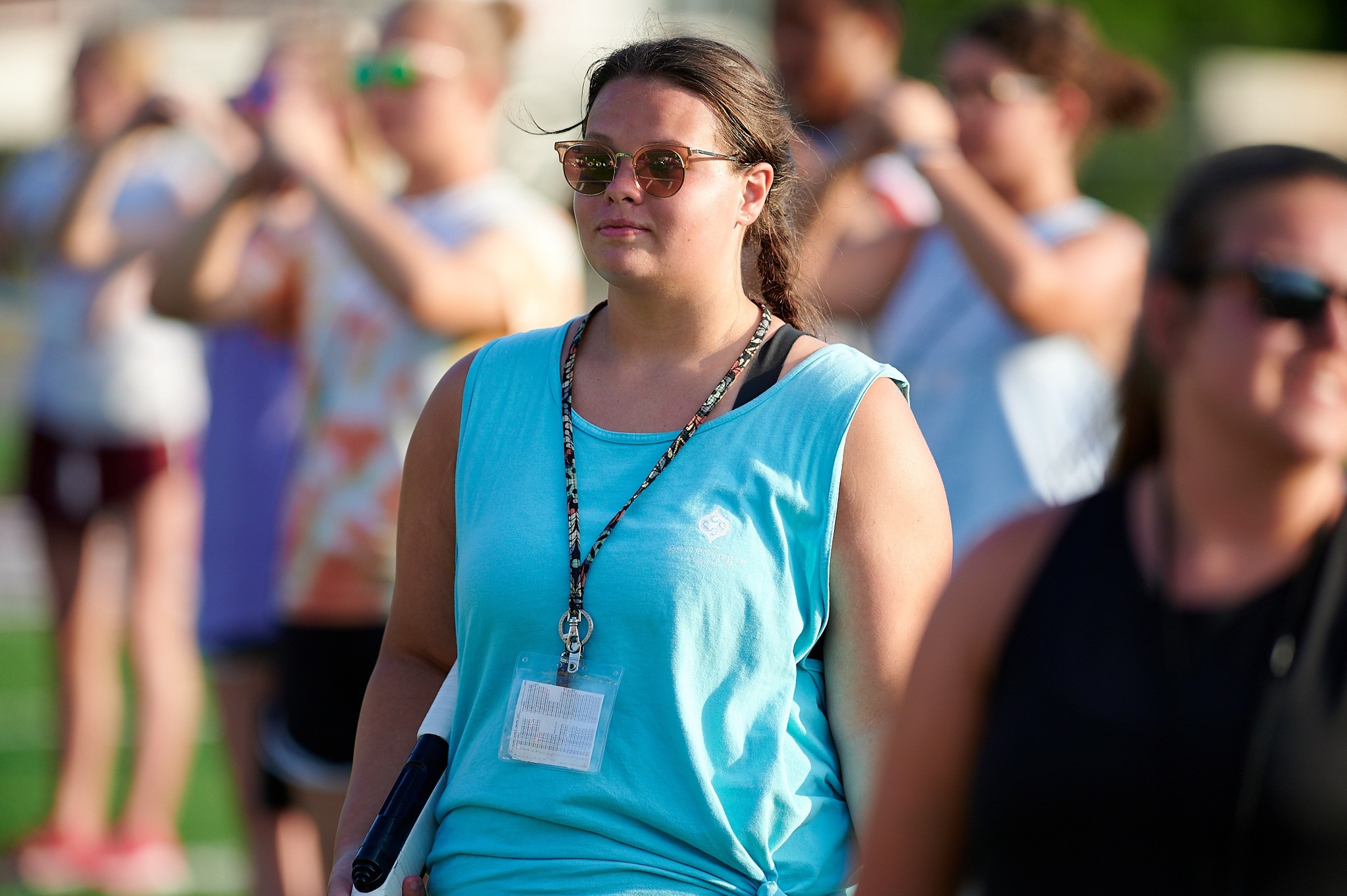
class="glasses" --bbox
[1183,255,1331,325]
[551,139,748,199]
[349,50,430,90]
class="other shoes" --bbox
[12,832,191,896]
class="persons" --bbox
[770,10,1169,577]
[326,42,952,895]
[0,2,584,895]
[858,146,1347,895]
